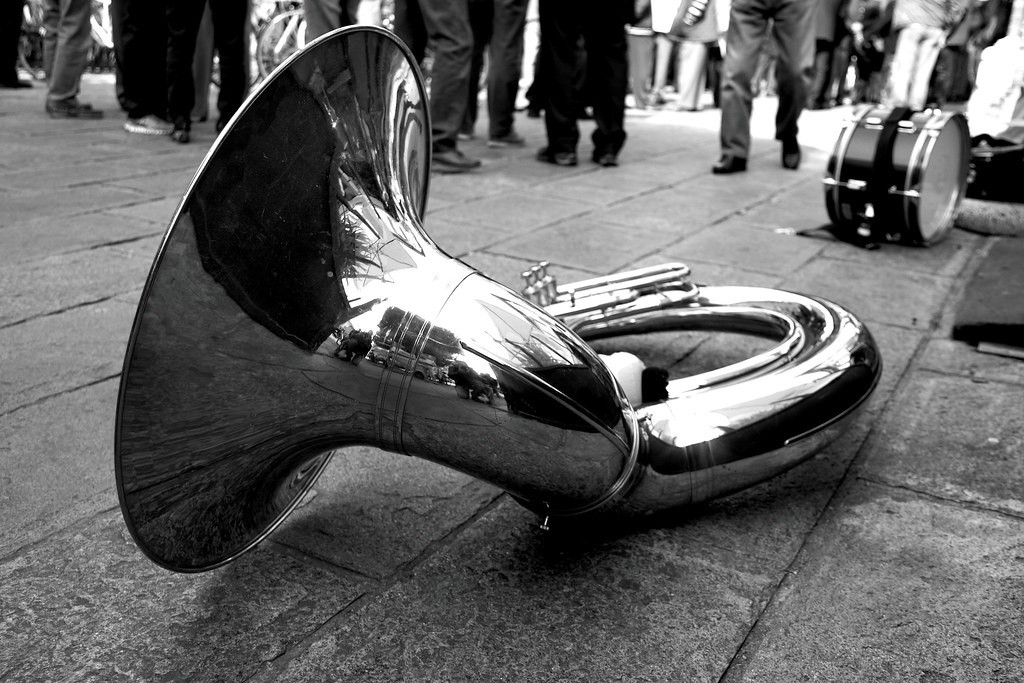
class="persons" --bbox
[301,0,636,175]
[0,0,250,174]
[648,0,1024,175]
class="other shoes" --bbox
[0,82,31,88]
[537,146,576,166]
[592,148,616,166]
[172,129,189,143]
[123,114,174,135]
[486,133,526,148]
[46,98,103,117]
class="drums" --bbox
[823,101,971,247]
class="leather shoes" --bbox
[782,138,800,170]
[430,148,481,172]
[712,155,745,173]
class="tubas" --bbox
[111,23,886,577]
